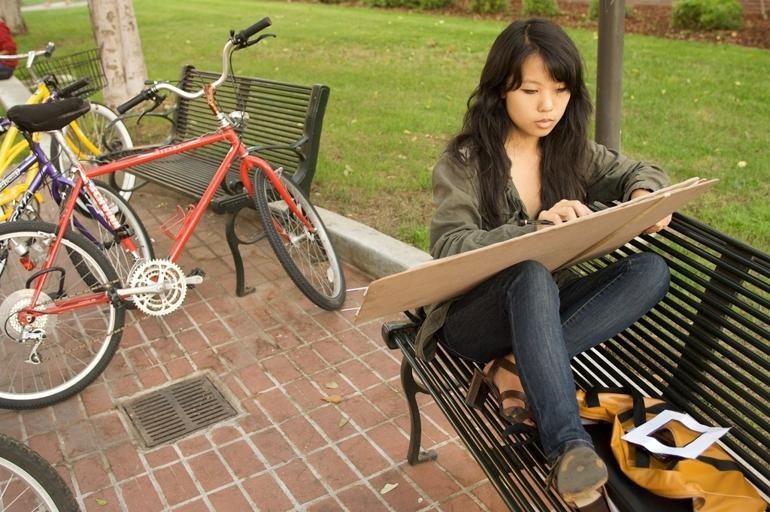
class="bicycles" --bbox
[0,16,346,410]
[0,77,156,310]
[0,41,135,258]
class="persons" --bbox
[414,16,675,508]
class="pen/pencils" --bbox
[518,219,569,226]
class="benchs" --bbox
[381,197,770,512]
[95,66,331,298]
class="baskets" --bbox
[13,48,108,101]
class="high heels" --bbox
[465,357,537,431]
[545,441,608,511]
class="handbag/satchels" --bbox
[576,384,768,511]
